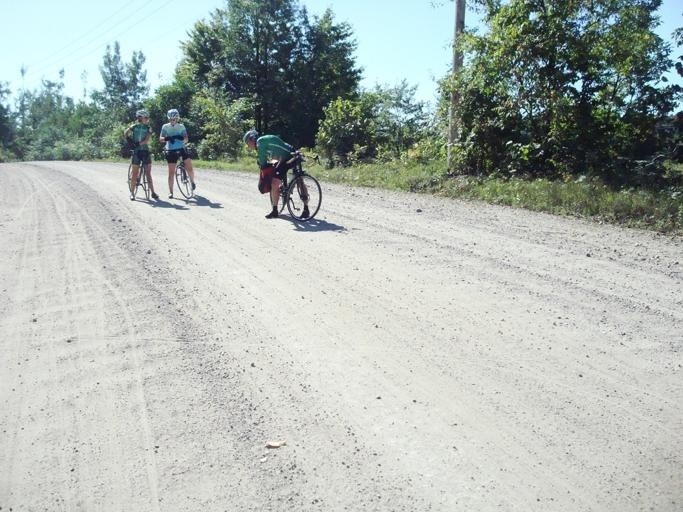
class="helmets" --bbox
[136,110,150,118]
[167,109,179,119]
[243,129,258,144]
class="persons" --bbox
[243,130,310,220]
[159,109,196,198]
[124,110,159,200]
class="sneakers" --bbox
[300,210,309,219]
[130,194,135,200]
[169,192,173,198]
[191,182,195,189]
[265,210,278,218]
[152,193,158,198]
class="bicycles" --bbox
[269,149,322,222]
[127,148,156,200]
[160,142,195,199]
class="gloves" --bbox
[126,136,140,150]
[165,135,184,144]
